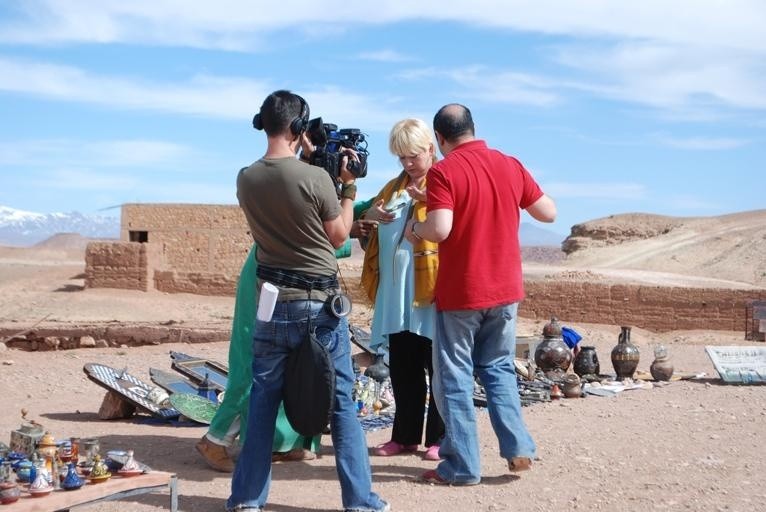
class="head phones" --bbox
[253,90,310,141]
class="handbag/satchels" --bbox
[283,333,337,436]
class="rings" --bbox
[379,216,384,220]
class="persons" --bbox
[358,118,446,462]
[195,197,379,473]
[224,90,391,512]
[403,103,557,485]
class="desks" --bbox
[0,454,184,512]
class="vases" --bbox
[649,357,673,381]
[612,324,639,383]
[363,352,390,383]
[571,345,601,377]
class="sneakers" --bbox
[224,499,264,512]
[423,469,480,485]
[425,444,443,460]
[346,499,390,512]
[509,455,532,472]
[375,438,419,456]
[271,448,316,462]
[195,435,233,473]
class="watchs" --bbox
[411,222,422,240]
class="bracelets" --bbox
[341,184,357,201]
